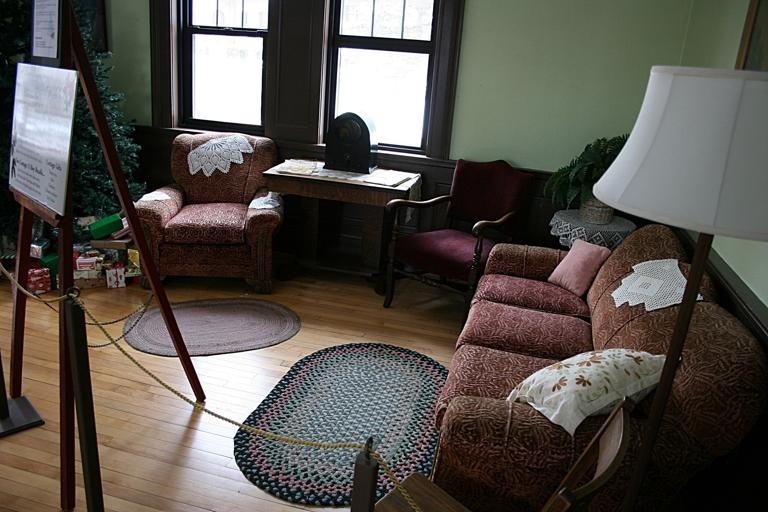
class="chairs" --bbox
[379,157,520,314]
[376,394,633,512]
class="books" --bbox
[363,168,410,188]
[290,157,324,172]
[276,158,315,174]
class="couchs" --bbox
[432,222,766,510]
[131,130,285,289]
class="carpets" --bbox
[232,342,451,510]
[123,296,301,357]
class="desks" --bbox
[550,208,637,251]
[259,157,421,293]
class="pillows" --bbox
[547,238,611,299]
[506,348,666,437]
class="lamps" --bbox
[589,62,767,445]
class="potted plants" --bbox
[542,132,628,226]
